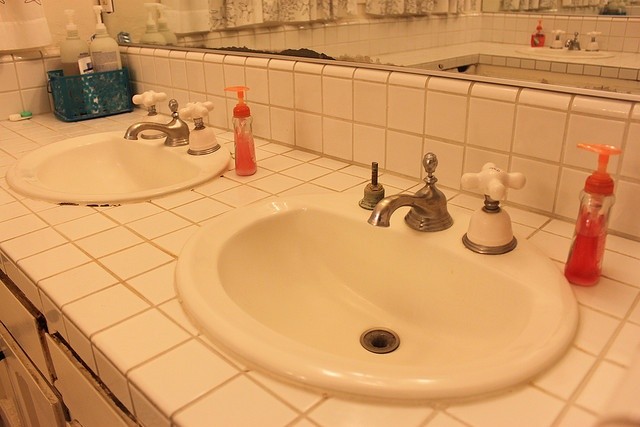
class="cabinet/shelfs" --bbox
[44,321,144,427]
[0,276,68,426]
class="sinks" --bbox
[176,204,579,402]
[5,132,231,203]
[515,46,615,59]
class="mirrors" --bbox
[99,0,640,104]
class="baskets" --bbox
[46,67,134,122]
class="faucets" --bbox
[124,99,189,147]
[565,31,580,50]
[368,153,453,231]
